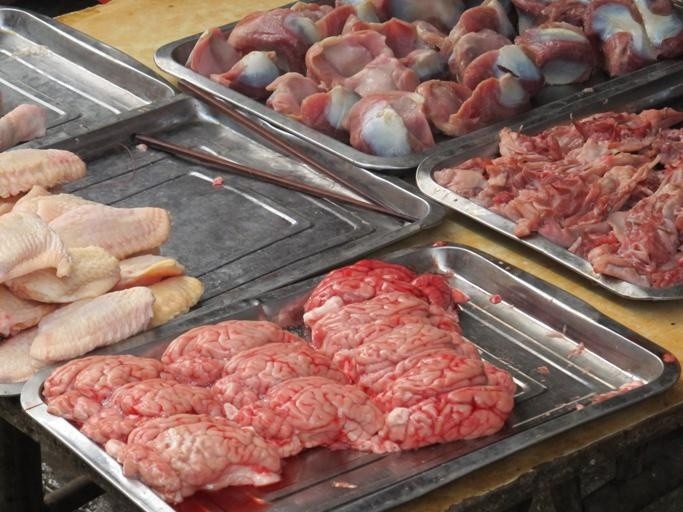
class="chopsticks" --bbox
[132,77,420,225]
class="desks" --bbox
[0,0,683,512]
